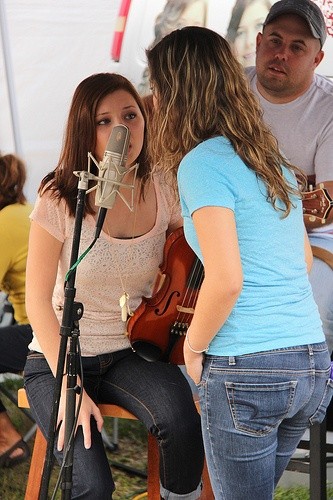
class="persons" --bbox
[145,27,333,500]
[243,0,333,357]
[0,154,35,468]
[23,72,204,500]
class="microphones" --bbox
[95,123,131,239]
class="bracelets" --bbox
[186,326,209,353]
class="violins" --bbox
[128,225,221,367]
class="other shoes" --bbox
[0,438,31,468]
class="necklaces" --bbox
[95,174,145,322]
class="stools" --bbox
[17,388,215,500]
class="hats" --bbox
[262,0,328,49]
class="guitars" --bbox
[298,181,333,224]
